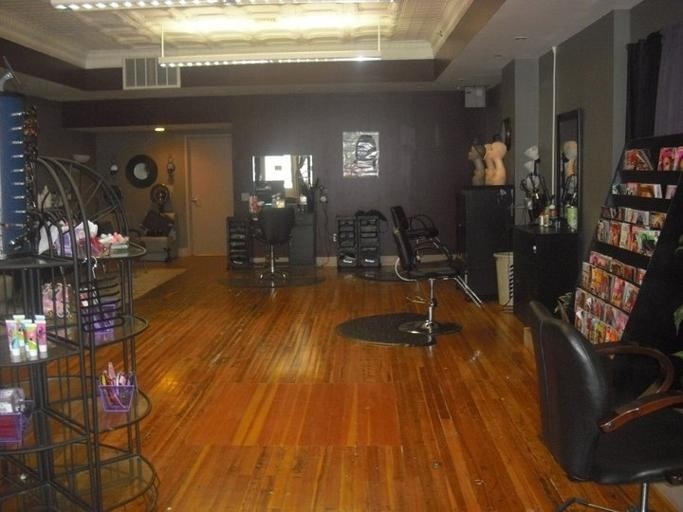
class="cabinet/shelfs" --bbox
[571,133,682,347]
[337,215,381,269]
[0,235,156,510]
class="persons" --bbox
[467,141,508,185]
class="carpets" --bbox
[125,268,188,304]
[334,312,435,347]
[352,266,415,282]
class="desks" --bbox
[511,224,576,327]
[226,212,318,274]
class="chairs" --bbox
[252,207,295,283]
[391,205,468,338]
[526,299,681,510]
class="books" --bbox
[575,146,683,360]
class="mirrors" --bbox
[557,109,583,233]
[251,153,313,207]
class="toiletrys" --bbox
[6,315,48,363]
